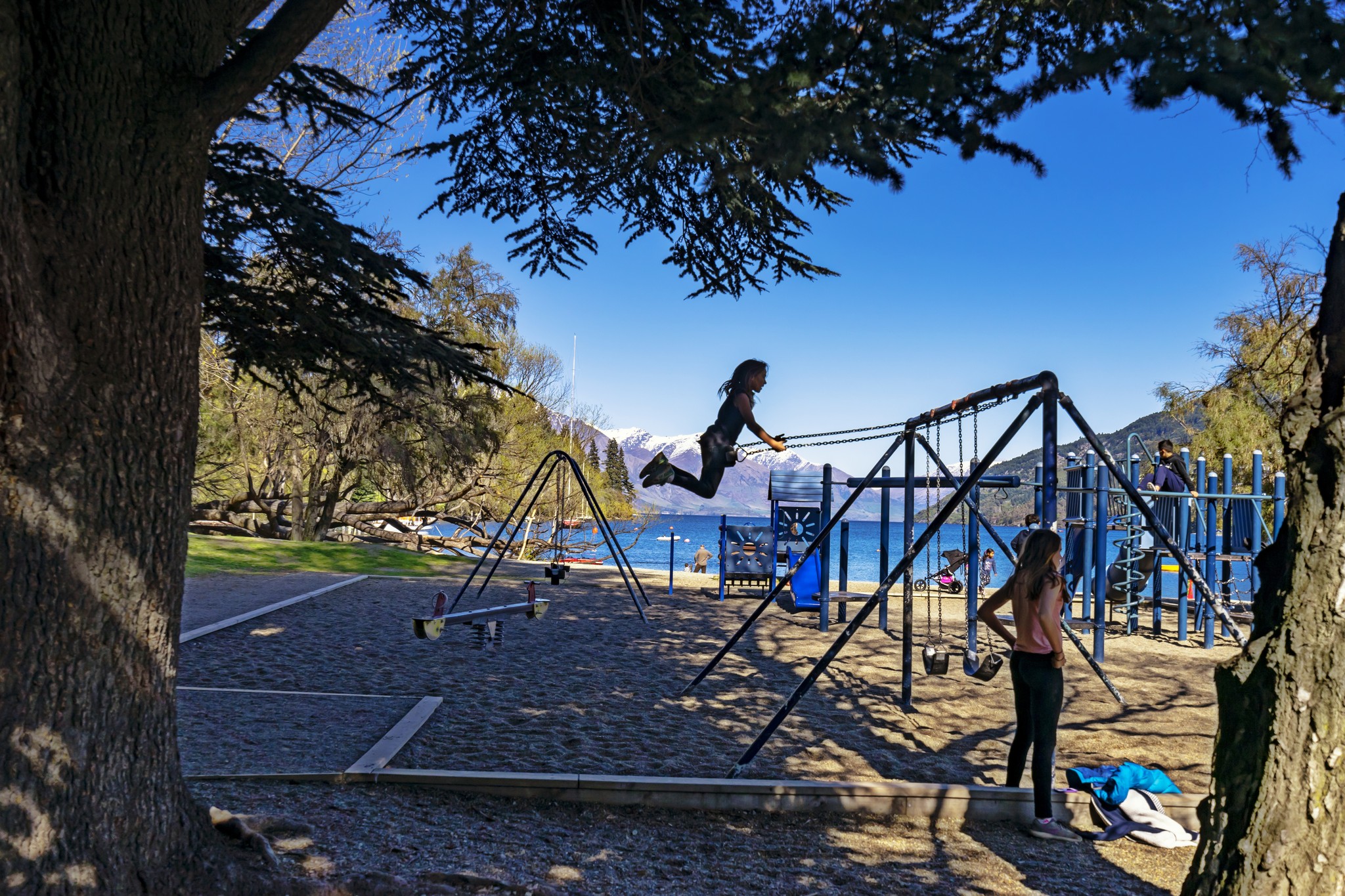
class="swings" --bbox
[921,418,950,676]
[543,460,575,587]
[701,396,1004,470]
[953,409,1006,682]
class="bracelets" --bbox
[1053,656,1065,662]
[1052,648,1063,655]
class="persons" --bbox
[1011,513,1041,559]
[784,519,798,586]
[1135,439,1199,501]
[975,529,1082,843]
[979,548,998,595]
[683,563,692,573]
[693,544,713,574]
[640,359,787,500]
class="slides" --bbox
[1090,530,1164,605]
[1055,521,1099,618]
[785,545,825,608]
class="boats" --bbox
[554,552,603,565]
[558,333,592,529]
[1057,530,1063,532]
[372,496,433,534]
[656,535,680,542]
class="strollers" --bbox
[914,547,968,594]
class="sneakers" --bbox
[1029,818,1083,842]
[980,590,987,595]
[639,451,669,480]
[642,463,674,489]
[978,586,981,592]
[1147,482,1159,501]
